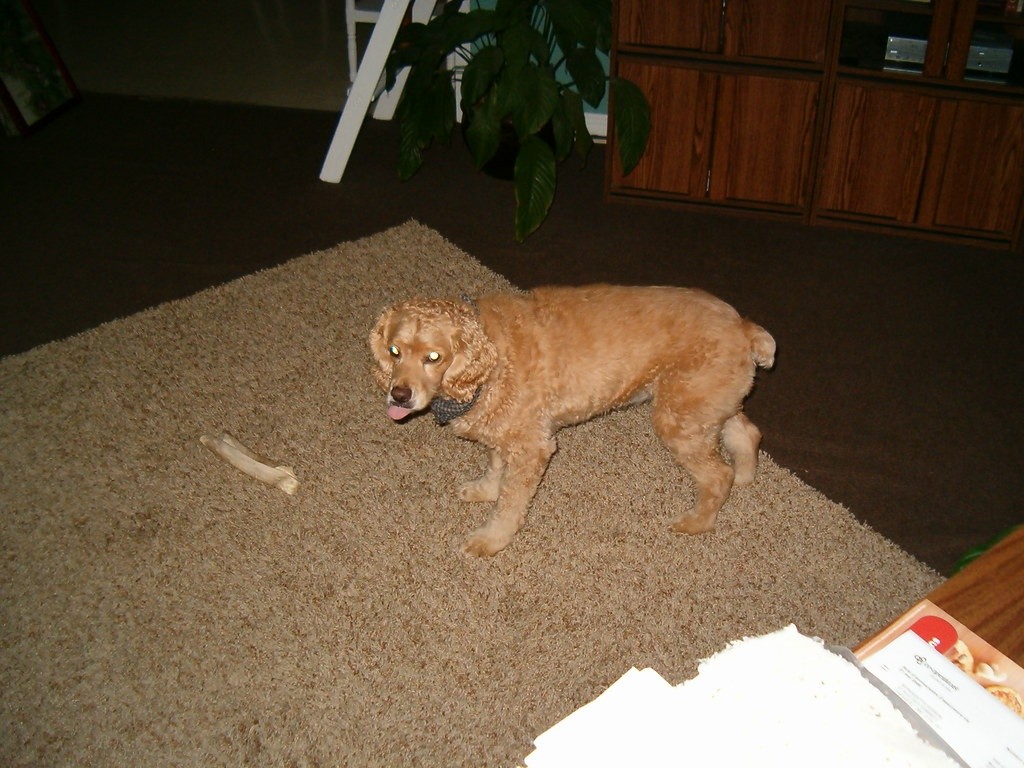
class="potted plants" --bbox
[386,0,652,242]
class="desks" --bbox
[847,524,1024,768]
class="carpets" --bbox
[0,216,947,768]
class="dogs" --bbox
[365,281,778,561]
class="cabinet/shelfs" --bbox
[604,0,1024,251]
